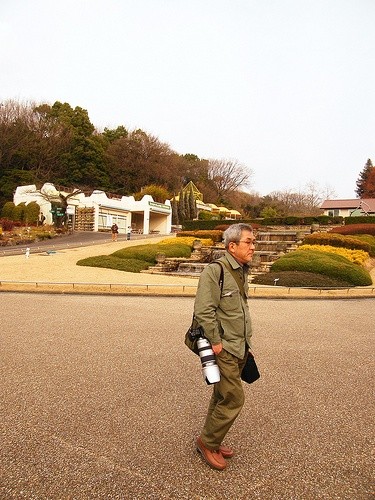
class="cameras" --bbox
[189,327,221,385]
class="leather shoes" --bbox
[196,436,234,470]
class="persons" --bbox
[41,212,45,225]
[192,223,256,471]
[127,226,132,240]
[111,223,118,242]
[341,219,345,227]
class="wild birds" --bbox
[46,250,56,255]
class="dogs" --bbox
[25,248,30,259]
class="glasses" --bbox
[230,240,258,247]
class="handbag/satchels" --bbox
[241,352,261,384]
[184,313,224,356]
